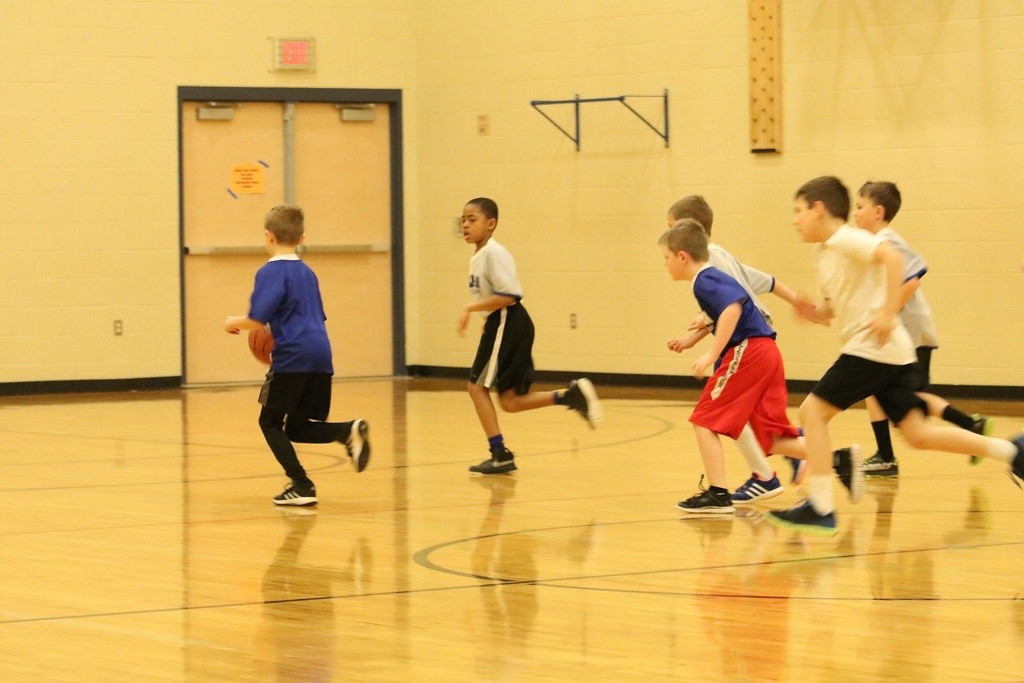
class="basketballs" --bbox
[250,322,275,363]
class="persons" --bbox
[456,197,604,476]
[223,204,370,506]
[768,176,1024,533]
[675,471,990,610]
[258,506,375,682]
[665,176,990,504]
[656,216,862,515]
[467,469,595,666]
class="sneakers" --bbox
[830,443,869,506]
[730,469,787,504]
[762,495,843,539]
[782,426,810,485]
[271,477,319,506]
[965,413,994,467]
[674,473,737,514]
[1006,432,1024,490]
[343,418,370,473]
[565,377,603,432]
[861,450,899,478]
[468,447,519,477]
